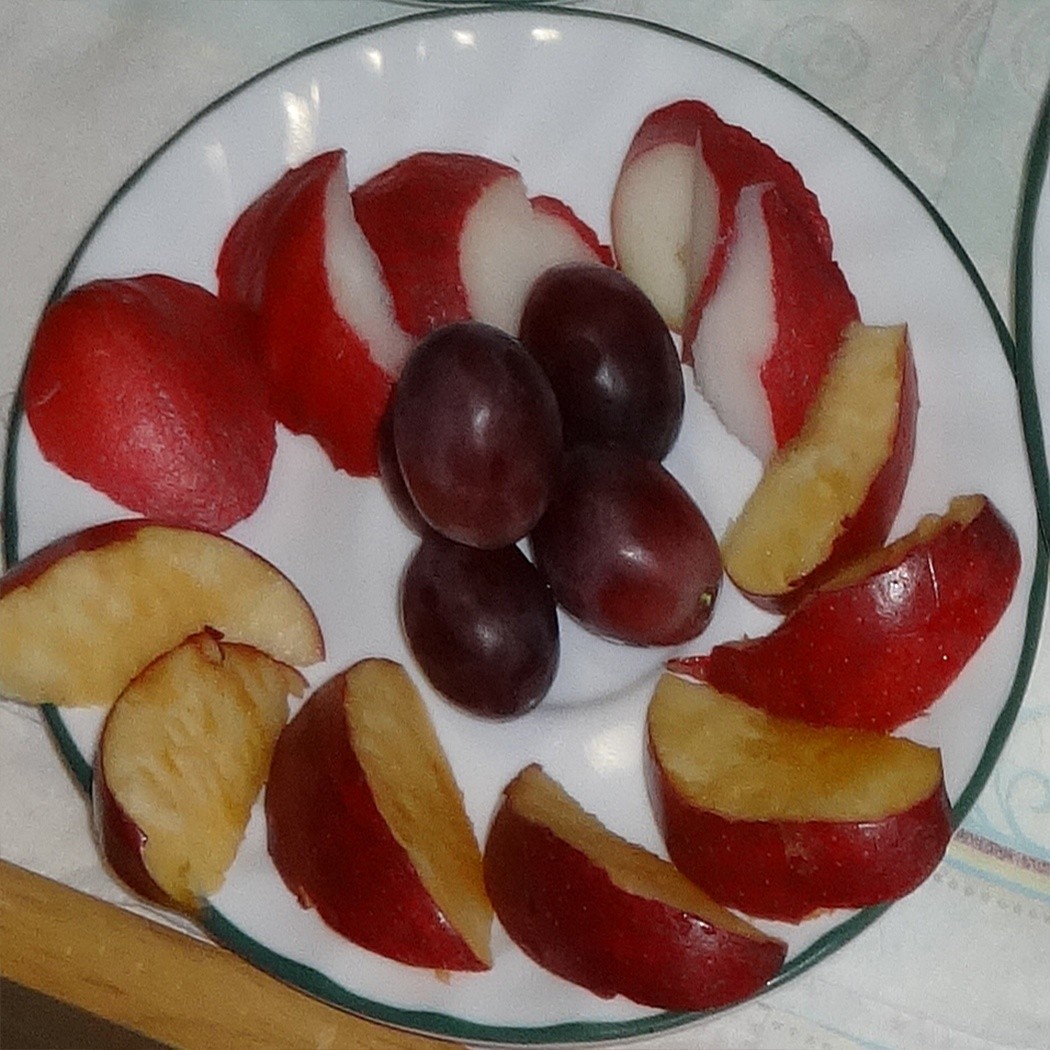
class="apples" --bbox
[0,518,327,910]
[267,657,495,971]
[665,495,1021,734]
[482,765,787,1013]
[646,673,953,924]
[718,323,918,611]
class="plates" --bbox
[2,3,1050,1046]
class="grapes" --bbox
[374,263,724,721]
